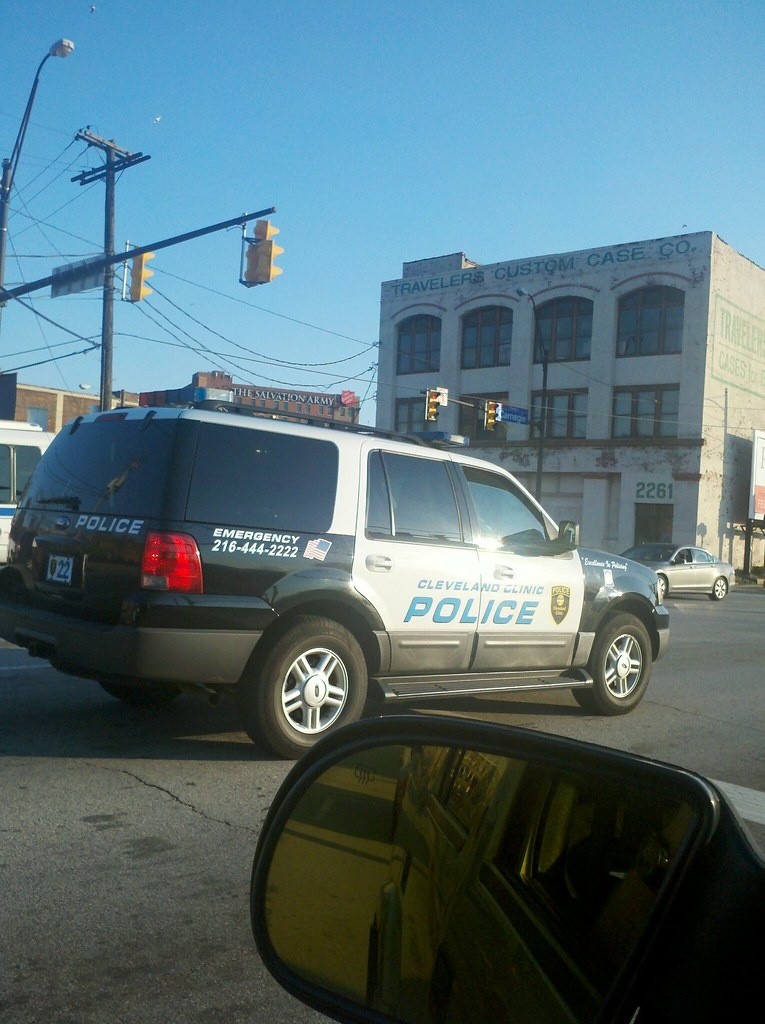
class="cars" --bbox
[618,542,738,602]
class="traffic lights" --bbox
[484,400,498,431]
[246,220,285,284]
[129,252,155,301]
[424,389,441,422]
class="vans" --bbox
[0,418,58,564]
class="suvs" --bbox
[0,399,671,760]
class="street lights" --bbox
[0,37,73,304]
[516,286,548,506]
